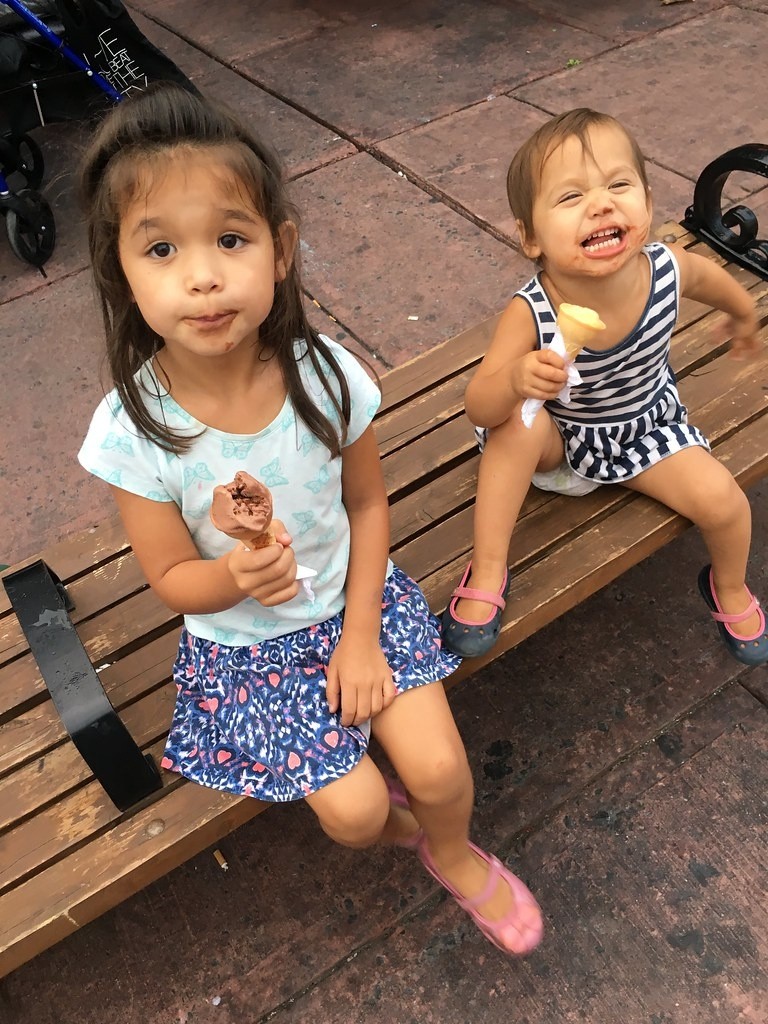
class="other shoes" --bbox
[418,838,546,960]
[386,780,435,859]
[440,561,512,659]
[697,564,768,667]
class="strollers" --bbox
[0,0,203,266]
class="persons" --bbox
[77,85,545,958]
[437,109,768,665]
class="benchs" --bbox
[0,142,768,976]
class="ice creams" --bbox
[557,303,606,364]
[210,471,276,553]
